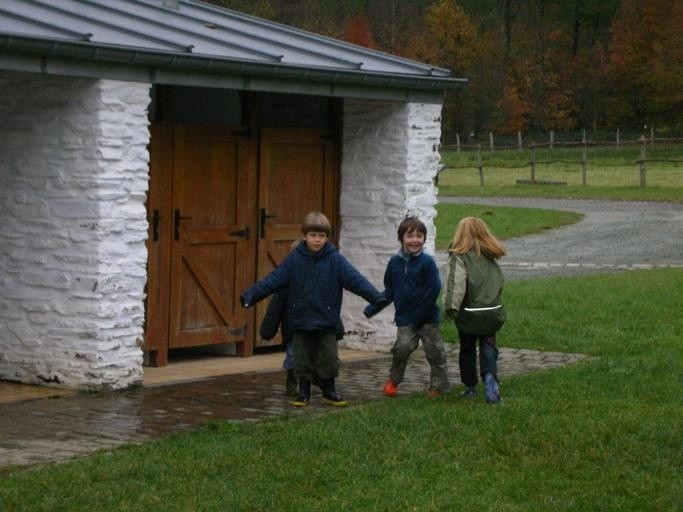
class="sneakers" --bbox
[430,389,441,398]
[286,386,296,396]
[461,388,479,397]
[384,378,399,396]
[484,372,501,404]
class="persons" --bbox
[362,216,453,399]
[440,214,508,404]
[238,210,390,406]
[258,238,345,395]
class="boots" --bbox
[320,378,347,406]
[290,380,311,407]
